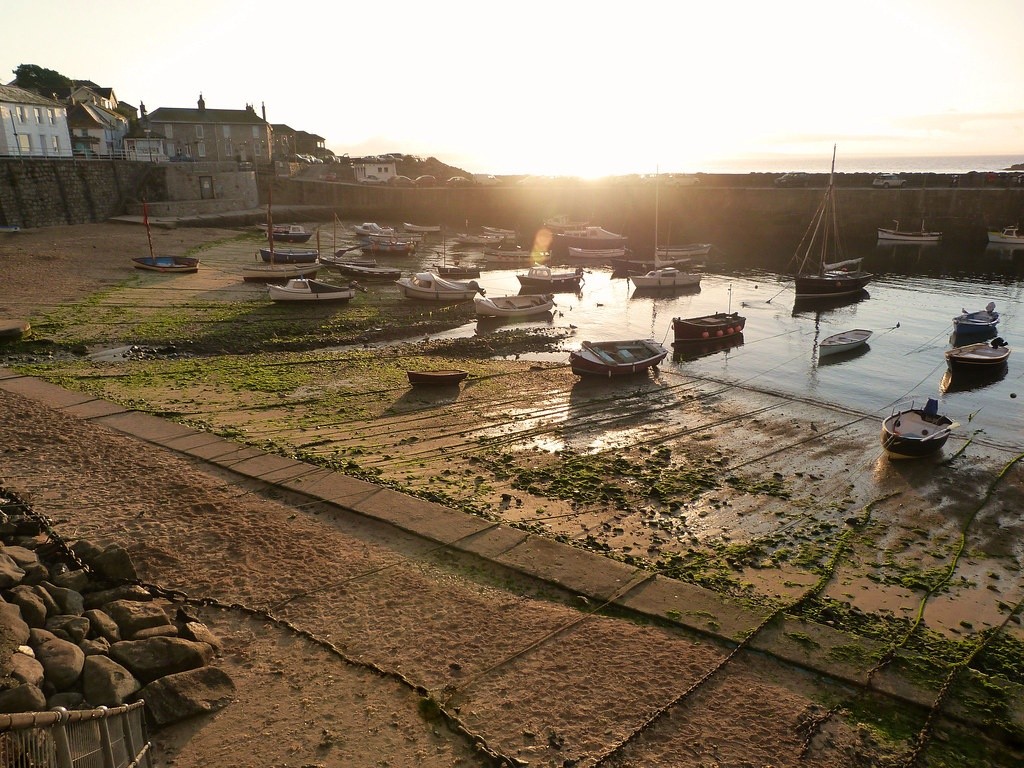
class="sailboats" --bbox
[795,140,873,296]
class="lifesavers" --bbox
[390,236,398,245]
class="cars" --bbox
[357,174,542,187]
[666,177,700,187]
[872,174,908,189]
[774,173,812,189]
[290,154,403,165]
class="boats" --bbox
[987,226,1024,243]
[880,399,955,456]
[944,301,1011,370]
[610,162,713,269]
[242,214,702,317]
[568,283,747,375]
[819,330,874,358]
[131,197,200,272]
[407,370,470,384]
[877,220,942,240]
[254,222,312,243]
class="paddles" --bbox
[920,421,962,444]
